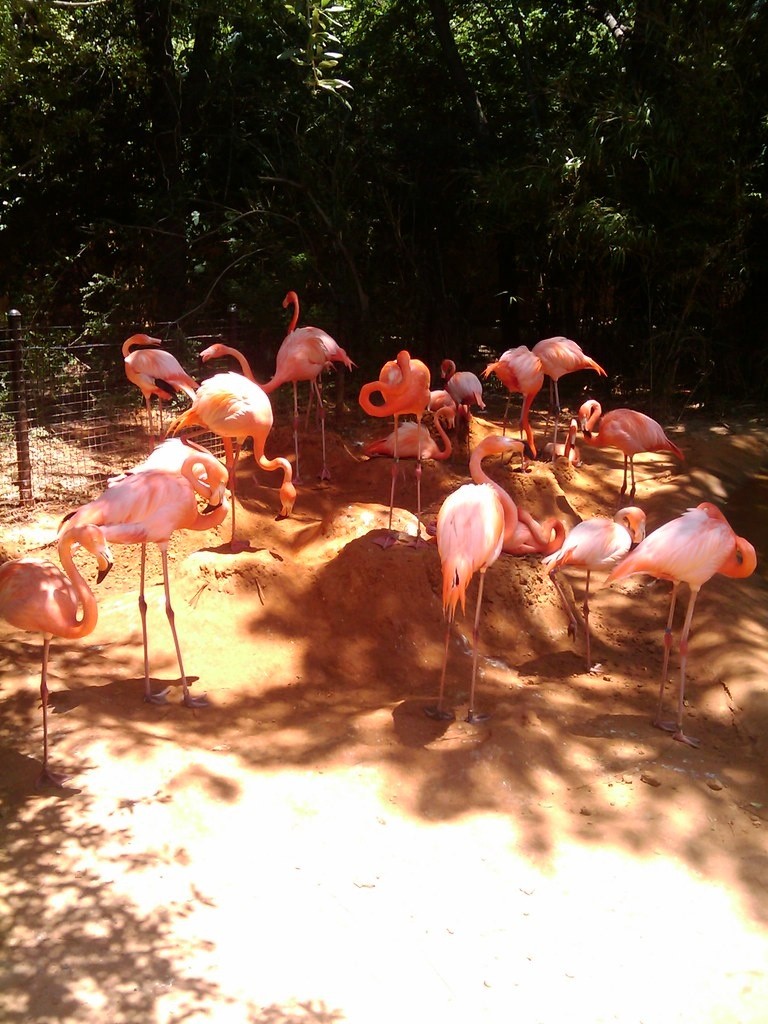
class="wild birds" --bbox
[0,521,115,796]
[0,286,759,751]
